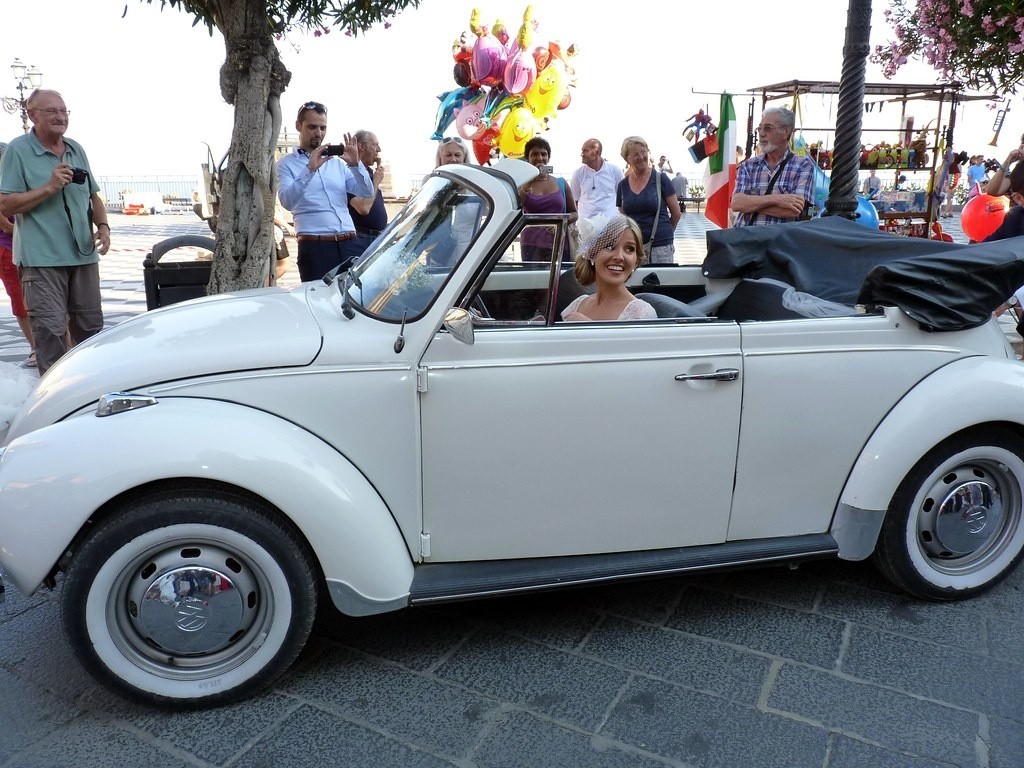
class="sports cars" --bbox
[0,160,1024,712]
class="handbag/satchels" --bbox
[566,223,581,261]
[638,240,652,265]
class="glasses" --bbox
[628,150,650,159]
[33,107,70,117]
[441,136,462,143]
[758,124,788,133]
[298,103,328,117]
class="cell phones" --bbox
[323,145,344,155]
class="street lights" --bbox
[0,56,44,137]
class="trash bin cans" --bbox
[142,234,216,313]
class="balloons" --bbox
[819,187,879,232]
[430,5,579,167]
[961,180,1012,243]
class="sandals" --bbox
[26,350,38,367]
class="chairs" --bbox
[540,267,596,322]
[632,293,708,322]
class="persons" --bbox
[735,145,744,164]
[731,109,813,231]
[0,89,110,377]
[529,214,659,321]
[864,152,1007,219]
[274,101,388,283]
[967,133,1024,360]
[421,136,688,268]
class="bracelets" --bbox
[998,164,1010,173]
[97,223,110,231]
[520,185,529,195]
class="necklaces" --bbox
[534,175,548,183]
[631,167,651,180]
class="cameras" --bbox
[540,166,553,173]
[68,167,87,185]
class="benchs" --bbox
[714,275,858,322]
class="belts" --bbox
[298,232,357,241]
[355,229,381,236]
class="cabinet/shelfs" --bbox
[745,78,962,237]
